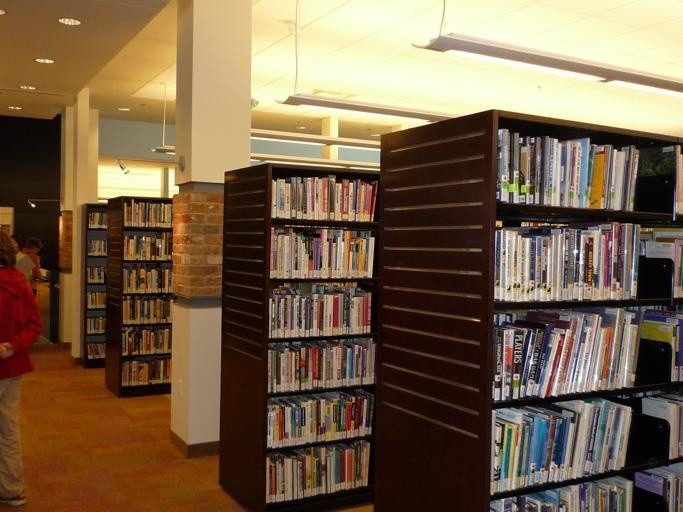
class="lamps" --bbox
[408,0,683,98]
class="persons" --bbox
[8,235,34,283]
[0,229,45,508]
[22,236,43,298]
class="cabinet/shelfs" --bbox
[76,196,172,398]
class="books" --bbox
[267,172,378,502]
[119,200,172,385]
[86,211,108,360]
[489,128,682,511]
